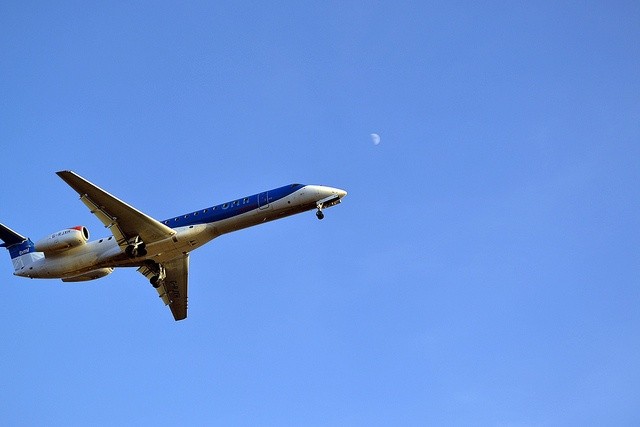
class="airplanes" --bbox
[0,170,348,321]
